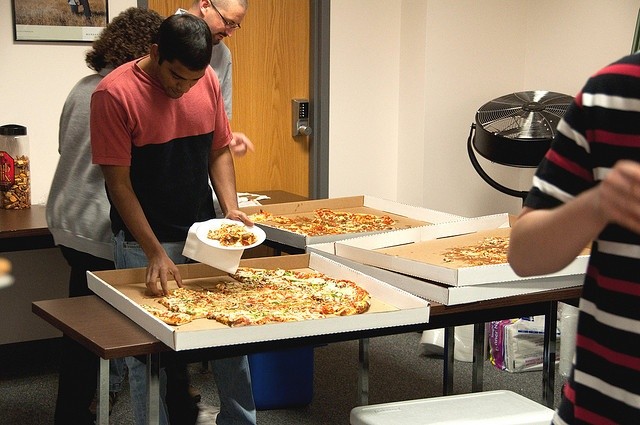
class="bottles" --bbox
[0,125,32,209]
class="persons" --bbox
[90,15,256,425]
[508,49,640,424]
[46,7,165,425]
[170,0,255,154]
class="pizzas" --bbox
[248,208,398,235]
[208,224,258,247]
[444,235,511,266]
[140,266,372,327]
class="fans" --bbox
[466,89,575,204]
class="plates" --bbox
[197,218,266,250]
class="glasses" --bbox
[209,0,241,29]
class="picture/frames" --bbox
[10,0,110,44]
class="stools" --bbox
[349,389,558,425]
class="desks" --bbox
[1,189,318,346]
[32,254,587,424]
